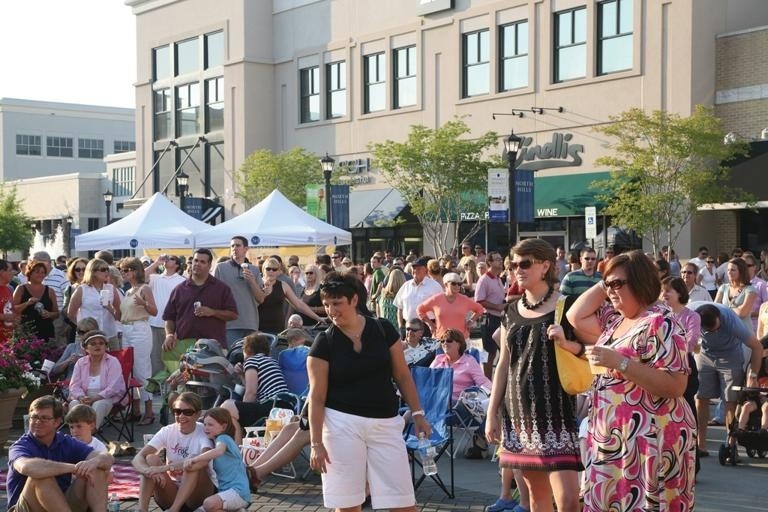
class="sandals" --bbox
[108,414,155,426]
[485,499,528,512]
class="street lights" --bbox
[30,221,36,255]
[503,129,521,250]
[65,214,74,257]
[320,151,335,223]
[101,190,112,226]
[174,169,189,213]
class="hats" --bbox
[82,330,107,344]
[410,257,426,266]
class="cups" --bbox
[194,301,202,316]
[40,359,55,380]
[100,290,110,306]
[584,345,614,376]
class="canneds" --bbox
[194,302,201,312]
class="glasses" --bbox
[172,409,196,416]
[603,279,630,290]
[511,258,545,269]
[407,327,424,332]
[332,256,339,258]
[448,281,463,286]
[78,331,85,335]
[266,267,278,272]
[584,257,596,260]
[28,415,54,422]
[682,252,754,274]
[306,271,314,275]
[440,339,453,342]
[60,260,135,272]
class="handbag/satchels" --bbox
[554,340,596,396]
[464,432,489,459]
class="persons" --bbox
[1,233,767,511]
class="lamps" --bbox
[723,128,768,145]
[492,106,562,120]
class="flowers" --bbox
[0,314,47,394]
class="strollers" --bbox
[162,333,278,423]
[719,335,767,465]
[274,319,331,352]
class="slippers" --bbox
[708,420,726,426]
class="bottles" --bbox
[109,493,120,512]
[34,299,46,319]
[694,334,702,355]
[417,432,438,475]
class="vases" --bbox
[0,387,26,430]
